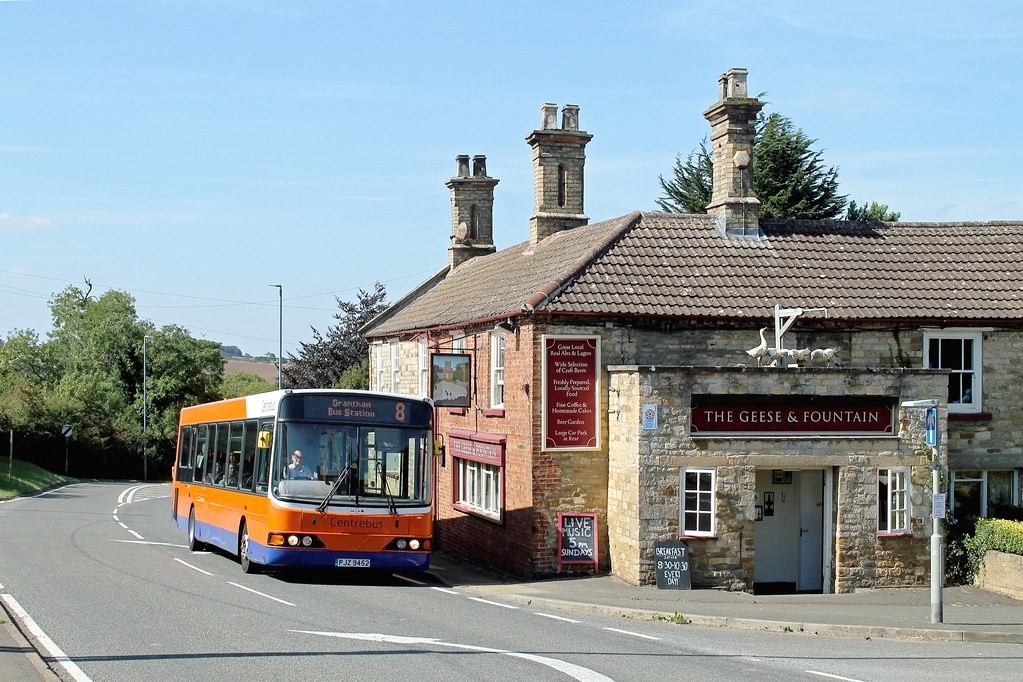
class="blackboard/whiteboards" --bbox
[557,512,598,563]
[653,541,691,590]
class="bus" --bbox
[170,388,446,575]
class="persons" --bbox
[206,462,223,483]
[283,448,318,481]
[218,462,239,487]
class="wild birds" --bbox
[745,326,768,366]
[765,346,842,368]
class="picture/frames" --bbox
[771,470,793,484]
[754,505,763,521]
[763,492,775,516]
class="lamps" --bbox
[492,321,521,335]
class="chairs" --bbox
[198,460,255,490]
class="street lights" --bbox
[269,284,283,390]
[899,398,944,623]
[143,335,154,481]
